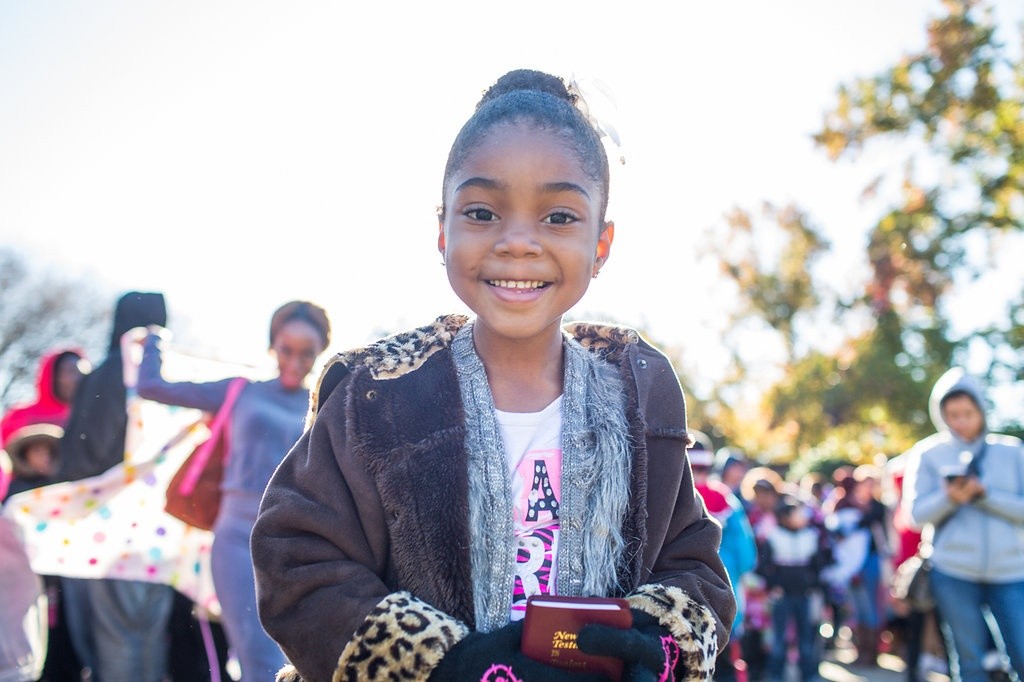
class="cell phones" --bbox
[945,471,965,485]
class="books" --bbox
[521,593,633,682]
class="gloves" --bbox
[428,593,681,682]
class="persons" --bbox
[133,300,331,681]
[671,425,926,682]
[243,68,734,682]
[900,363,1023,682]
[1,290,198,682]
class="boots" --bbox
[846,626,880,669]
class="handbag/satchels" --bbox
[886,552,936,616]
[163,429,229,535]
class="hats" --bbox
[686,427,714,475]
